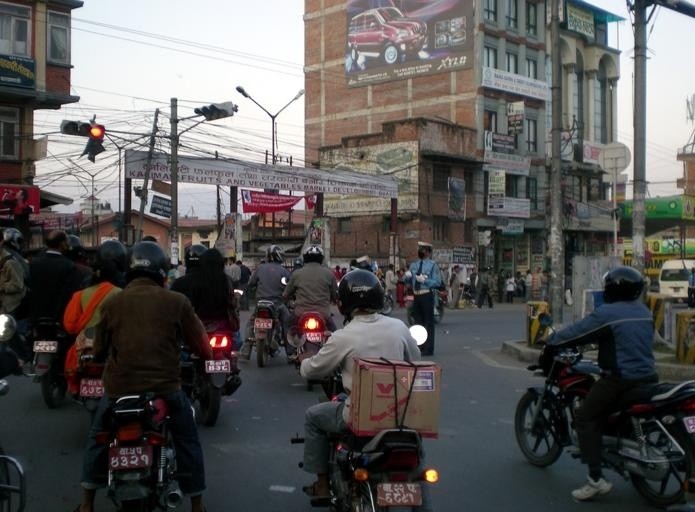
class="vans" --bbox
[658,258,695,303]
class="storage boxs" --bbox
[349,356,442,439]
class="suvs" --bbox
[349,5,429,64]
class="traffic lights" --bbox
[58,119,106,140]
[193,99,234,123]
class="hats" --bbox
[417,241,433,251]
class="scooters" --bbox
[0,313,26,512]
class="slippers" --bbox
[303,480,331,498]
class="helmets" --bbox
[266,245,285,264]
[603,267,643,304]
[69,235,83,254]
[303,246,323,264]
[338,270,383,315]
[185,246,206,267]
[4,229,24,252]
[100,241,170,285]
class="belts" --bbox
[413,290,430,295]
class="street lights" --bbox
[236,84,308,166]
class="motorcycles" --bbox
[191,290,243,428]
[243,286,286,369]
[286,325,439,511]
[99,345,203,511]
[15,313,106,417]
[511,310,695,511]
[281,276,340,393]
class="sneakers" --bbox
[287,355,296,362]
[572,475,613,500]
[238,352,249,360]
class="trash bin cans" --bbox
[677,309,695,362]
[527,300,549,348]
[648,292,665,340]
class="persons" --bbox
[545,266,659,501]
[280,246,340,367]
[353,256,374,272]
[76,240,207,511]
[223,257,252,311]
[239,245,296,364]
[2,228,241,401]
[682,259,695,309]
[390,244,441,355]
[384,263,549,310]
[2,188,35,227]
[300,270,433,510]
